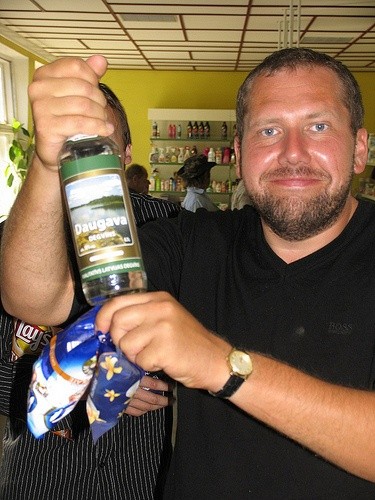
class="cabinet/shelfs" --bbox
[147,108,236,208]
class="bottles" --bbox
[192,121,198,138]
[55,134,148,307]
[152,121,157,137]
[176,125,181,139]
[221,122,227,137]
[187,121,192,139]
[198,121,204,138]
[149,145,235,163]
[168,124,172,139]
[204,121,210,138]
[172,124,176,139]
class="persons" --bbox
[1,84,192,498]
[177,154,218,212]
[231,179,253,210]
[1,47,375,499]
[125,163,148,193]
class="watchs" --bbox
[209,341,254,400]
